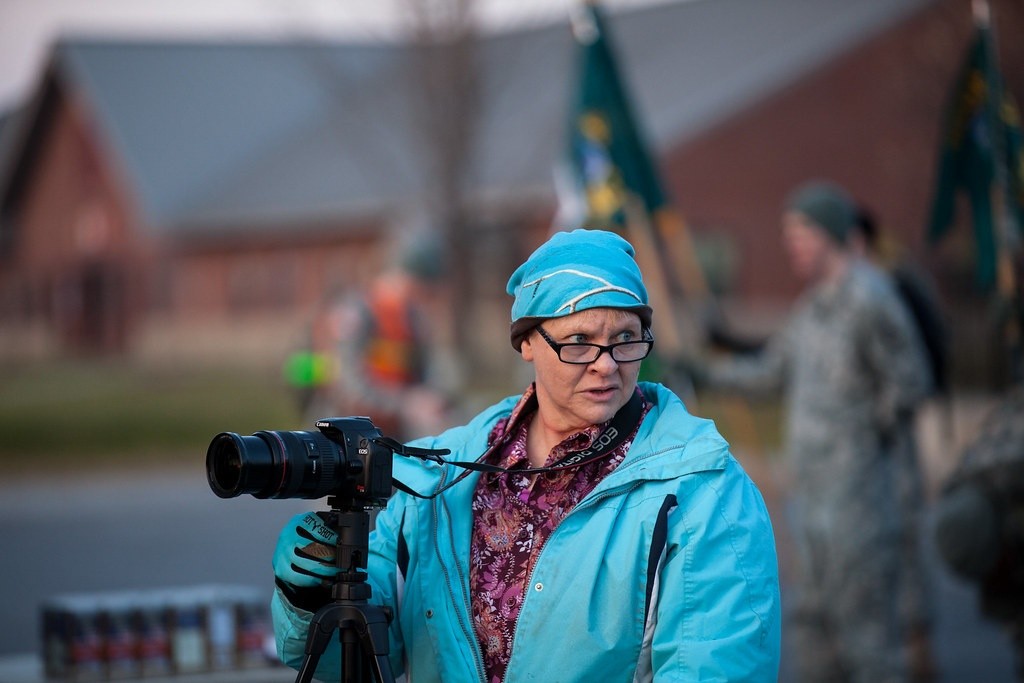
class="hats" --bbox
[506,228,653,355]
[798,190,855,246]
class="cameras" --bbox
[205,415,393,511]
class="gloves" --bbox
[270,511,341,610]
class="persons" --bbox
[269,227,783,682]
[688,198,954,683]
[666,193,943,683]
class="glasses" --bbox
[535,324,655,364]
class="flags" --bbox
[910,1,1023,371]
[544,3,695,417]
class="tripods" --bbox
[295,497,397,683]
